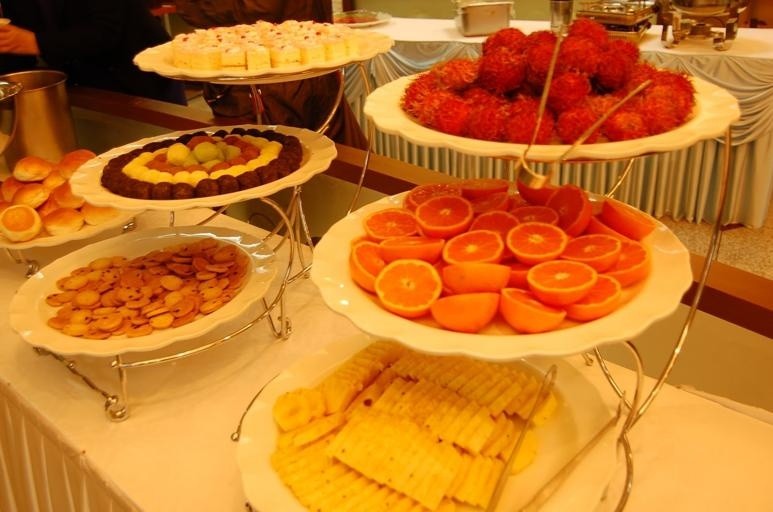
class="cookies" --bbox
[45,237,250,339]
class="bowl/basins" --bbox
[0,81,24,157]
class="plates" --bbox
[237,335,618,512]
[363,69,742,162]
[332,9,394,27]
[132,22,393,79]
[70,124,338,212]
[7,226,277,356]
[0,209,147,250]
[310,181,693,361]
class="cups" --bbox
[0,18,11,26]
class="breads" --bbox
[0,149,119,242]
[160,20,365,73]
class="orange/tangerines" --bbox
[350,178,657,332]
